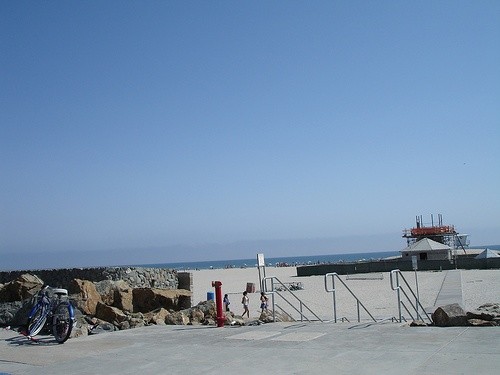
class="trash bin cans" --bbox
[207,291,215,301]
[246,282,255,293]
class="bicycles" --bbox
[23,280,74,344]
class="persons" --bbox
[224,293,231,309]
[185,257,384,289]
[260,292,269,314]
[241,291,250,319]
